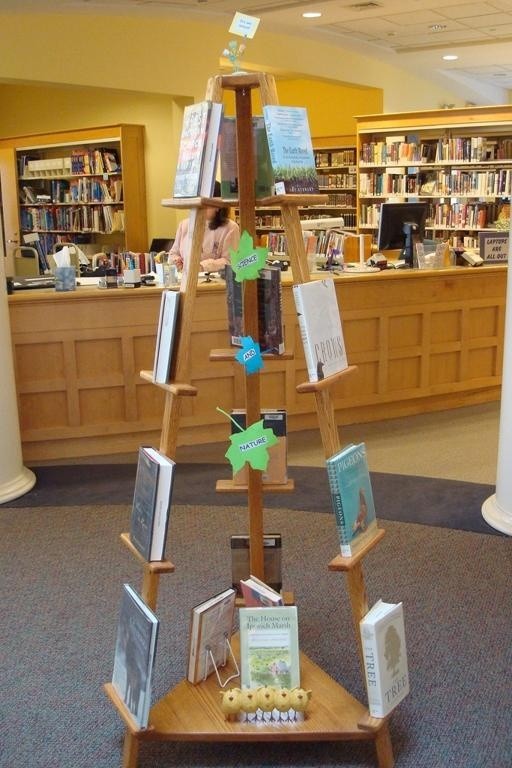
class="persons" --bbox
[169,180,240,272]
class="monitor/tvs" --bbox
[378,202,428,268]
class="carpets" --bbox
[0,507,512,768]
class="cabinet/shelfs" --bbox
[353,105,512,263]
[236,143,357,259]
[0,123,147,262]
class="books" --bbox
[240,605,300,691]
[292,277,349,384]
[19,147,168,287]
[360,131,511,248]
[240,575,283,607]
[172,99,357,257]
[186,587,236,685]
[128,446,177,563]
[231,533,282,597]
[112,583,160,730]
[224,264,247,349]
[257,265,285,355]
[152,290,181,384]
[230,409,288,484]
[327,442,378,558]
[359,599,409,718]
